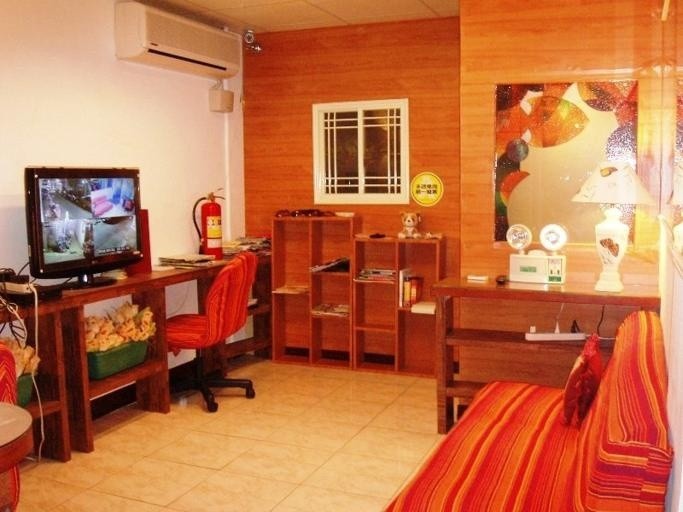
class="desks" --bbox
[1,402,35,512]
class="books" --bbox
[359,268,436,316]
[276,284,308,293]
[310,303,350,318]
[159,253,217,270]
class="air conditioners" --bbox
[114,2,240,81]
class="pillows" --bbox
[563,334,605,428]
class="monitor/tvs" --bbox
[23,167,144,289]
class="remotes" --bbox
[496,274,505,283]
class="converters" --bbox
[0,268,16,282]
[11,275,28,284]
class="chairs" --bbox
[167,252,258,412]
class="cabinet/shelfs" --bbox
[0,249,270,448]
[430,276,660,434]
[352,233,448,381]
[271,212,362,369]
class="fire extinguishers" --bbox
[192,188,226,260]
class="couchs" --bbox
[382,310,675,512]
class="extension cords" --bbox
[0,281,33,295]
[525,333,586,341]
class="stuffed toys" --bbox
[397,210,422,239]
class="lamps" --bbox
[570,161,656,292]
[505,223,569,283]
